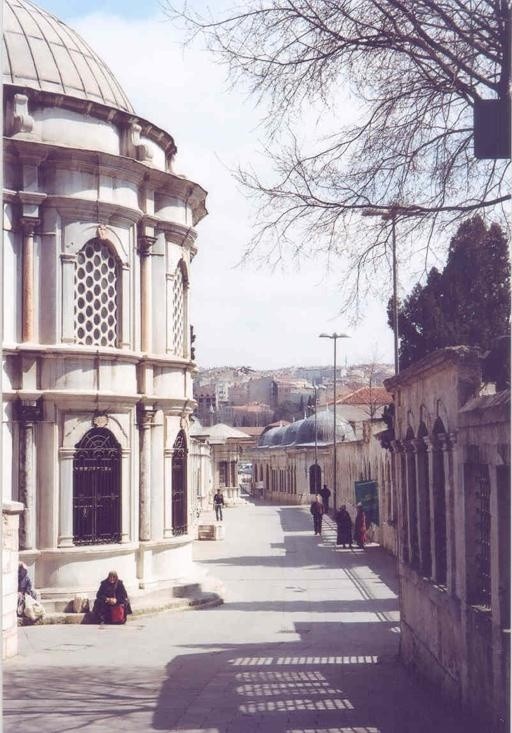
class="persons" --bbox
[351,502,367,548]
[213,488,225,521]
[319,484,331,513]
[92,570,133,624]
[16,562,34,616]
[310,494,323,535]
[334,504,353,548]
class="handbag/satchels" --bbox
[24,594,45,621]
[110,603,126,625]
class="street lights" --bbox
[361,209,425,375]
[319,333,348,521]
[304,385,326,494]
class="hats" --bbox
[354,502,363,507]
[109,571,118,577]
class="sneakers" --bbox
[342,544,367,550]
